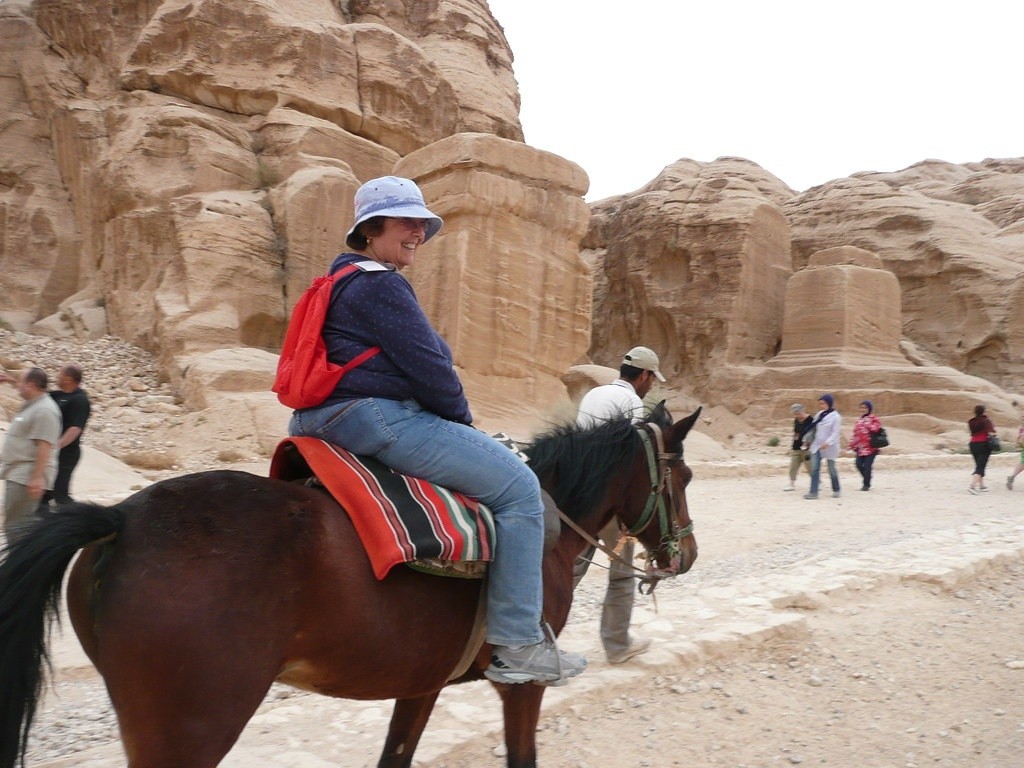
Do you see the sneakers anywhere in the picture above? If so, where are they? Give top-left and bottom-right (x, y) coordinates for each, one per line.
(484, 635), (588, 684)
(609, 637), (651, 663)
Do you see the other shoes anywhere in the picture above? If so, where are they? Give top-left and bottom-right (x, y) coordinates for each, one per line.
(833, 491), (839, 498)
(967, 485), (978, 495)
(1007, 476), (1014, 490)
(861, 482), (870, 491)
(783, 485), (795, 491)
(979, 486), (988, 492)
(804, 492), (818, 499)
(818, 483), (824, 489)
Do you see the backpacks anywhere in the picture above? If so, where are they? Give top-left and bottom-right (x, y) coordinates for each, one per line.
(271, 262), (382, 409)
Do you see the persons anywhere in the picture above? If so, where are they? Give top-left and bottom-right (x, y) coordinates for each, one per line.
(801, 394), (840, 500)
(572, 345), (653, 664)
(968, 405), (996, 495)
(287, 176), (587, 685)
(783, 403), (824, 491)
(0, 367), (62, 554)
(0, 364), (90, 506)
(1006, 425), (1024, 490)
(847, 400), (880, 491)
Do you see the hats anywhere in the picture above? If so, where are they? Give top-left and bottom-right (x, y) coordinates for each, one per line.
(861, 401), (872, 412)
(820, 394), (832, 408)
(345, 176), (443, 252)
(791, 404), (804, 414)
(623, 347), (666, 383)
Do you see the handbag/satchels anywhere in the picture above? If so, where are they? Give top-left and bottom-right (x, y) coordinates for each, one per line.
(987, 438), (1000, 451)
(871, 428), (889, 448)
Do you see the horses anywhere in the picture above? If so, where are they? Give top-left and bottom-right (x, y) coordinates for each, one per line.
(0, 398), (704, 768)
